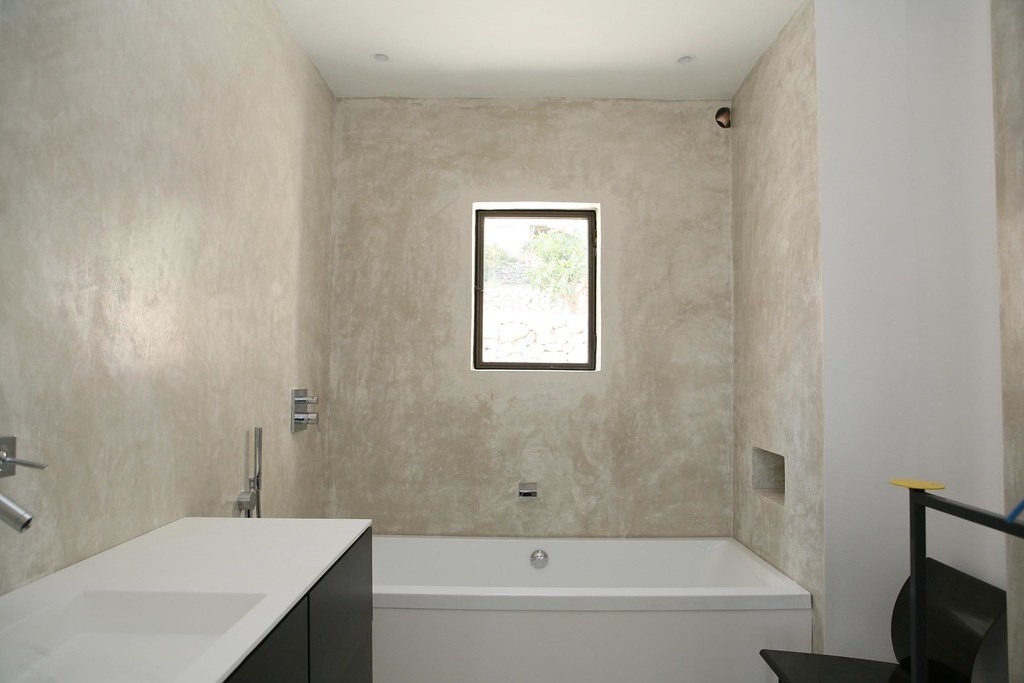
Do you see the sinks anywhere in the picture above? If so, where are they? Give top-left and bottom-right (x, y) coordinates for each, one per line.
(0, 588), (267, 683)
(111, 526), (331, 585)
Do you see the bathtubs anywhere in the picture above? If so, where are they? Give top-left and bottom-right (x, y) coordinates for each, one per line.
(373, 536), (811, 683)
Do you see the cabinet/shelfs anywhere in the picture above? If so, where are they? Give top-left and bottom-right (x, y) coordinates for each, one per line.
(225, 525), (373, 683)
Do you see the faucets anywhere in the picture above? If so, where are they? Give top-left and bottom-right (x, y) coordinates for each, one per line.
(0, 494), (34, 532)
